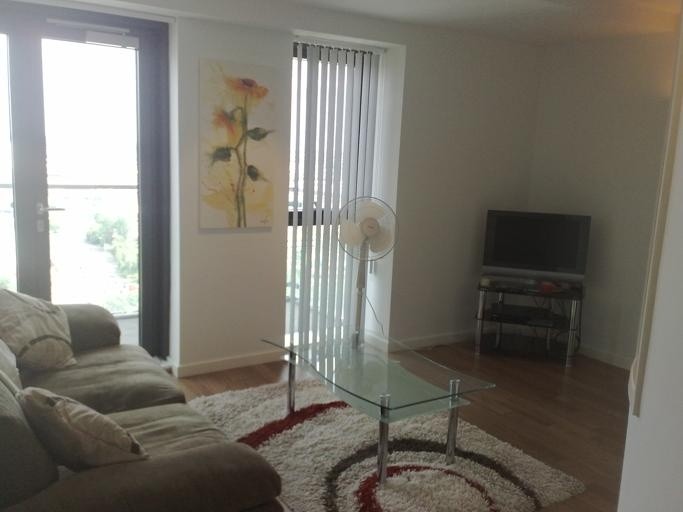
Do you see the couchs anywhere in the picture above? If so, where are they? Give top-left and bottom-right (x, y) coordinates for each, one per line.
(0, 287), (285, 512)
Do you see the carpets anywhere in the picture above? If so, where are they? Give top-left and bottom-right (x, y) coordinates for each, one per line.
(188, 375), (585, 512)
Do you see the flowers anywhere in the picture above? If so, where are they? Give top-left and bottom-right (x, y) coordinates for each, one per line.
(200, 76), (276, 229)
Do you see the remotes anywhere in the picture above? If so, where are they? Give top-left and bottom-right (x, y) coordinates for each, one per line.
(495, 287), (528, 294)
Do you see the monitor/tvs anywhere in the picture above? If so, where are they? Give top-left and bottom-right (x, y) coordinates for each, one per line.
(480, 209), (591, 293)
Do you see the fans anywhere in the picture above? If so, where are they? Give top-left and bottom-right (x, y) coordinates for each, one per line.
(334, 195), (400, 332)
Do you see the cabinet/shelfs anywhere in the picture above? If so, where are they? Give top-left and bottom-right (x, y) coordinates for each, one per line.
(471, 280), (584, 368)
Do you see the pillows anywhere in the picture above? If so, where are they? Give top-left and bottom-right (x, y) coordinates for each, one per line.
(0, 288), (78, 371)
(16, 385), (149, 472)
